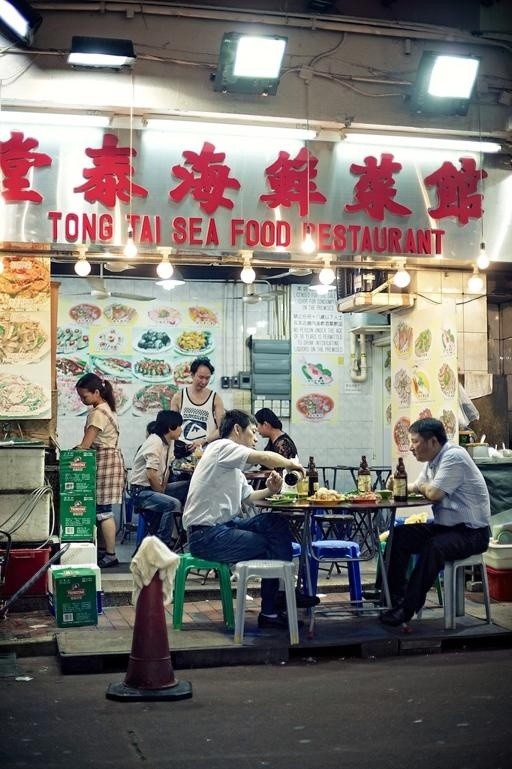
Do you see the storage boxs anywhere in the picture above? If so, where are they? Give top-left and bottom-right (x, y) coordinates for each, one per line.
(0, 449), (103, 627)
(481, 541), (512, 602)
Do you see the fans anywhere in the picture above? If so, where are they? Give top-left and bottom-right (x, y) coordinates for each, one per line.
(56, 263), (155, 302)
(217, 269), (286, 304)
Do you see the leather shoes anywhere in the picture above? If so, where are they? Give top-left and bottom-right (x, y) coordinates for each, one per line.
(258, 589), (320, 629)
(362, 588), (418, 626)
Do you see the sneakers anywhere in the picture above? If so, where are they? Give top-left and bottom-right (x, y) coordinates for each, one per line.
(97, 557), (119, 568)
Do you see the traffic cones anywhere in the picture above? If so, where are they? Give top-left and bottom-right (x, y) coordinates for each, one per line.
(103, 532), (195, 703)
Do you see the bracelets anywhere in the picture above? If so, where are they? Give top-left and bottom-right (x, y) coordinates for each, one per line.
(202, 436), (210, 443)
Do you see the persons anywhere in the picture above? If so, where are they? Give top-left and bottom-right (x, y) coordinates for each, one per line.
(131, 422), (203, 483)
(170, 355), (223, 468)
(130, 411), (194, 552)
(183, 408), (320, 630)
(248, 408), (299, 491)
(76, 373), (122, 566)
(375, 418), (489, 627)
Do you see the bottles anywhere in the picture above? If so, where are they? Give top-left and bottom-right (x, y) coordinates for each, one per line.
(306, 455), (318, 497)
(285, 469), (304, 485)
(358, 455), (371, 491)
(458, 429), (477, 452)
(394, 458), (408, 502)
(195, 444), (203, 469)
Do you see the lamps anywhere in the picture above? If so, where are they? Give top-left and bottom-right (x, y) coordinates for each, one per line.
(210, 31), (288, 95)
(124, 63), (137, 259)
(64, 36), (136, 70)
(0, 0), (44, 47)
(407, 50), (482, 117)
(466, 262), (485, 295)
(299, 65), (317, 254)
(475, 54), (490, 268)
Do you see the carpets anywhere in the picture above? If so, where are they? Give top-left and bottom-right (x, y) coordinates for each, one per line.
(427, 587), (512, 631)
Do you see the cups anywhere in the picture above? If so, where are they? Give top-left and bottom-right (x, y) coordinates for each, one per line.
(296, 477), (309, 500)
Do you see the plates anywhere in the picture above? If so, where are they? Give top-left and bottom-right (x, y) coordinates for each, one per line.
(0, 256), (221, 418)
(265, 490), (425, 504)
(297, 363), (336, 422)
(384, 325), (458, 455)
